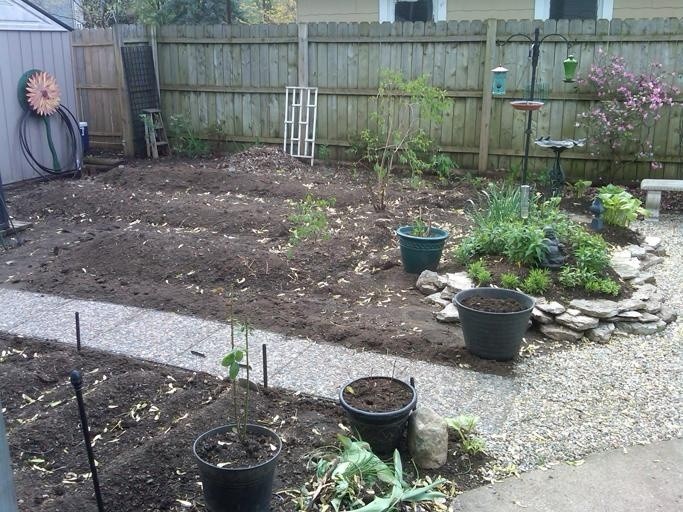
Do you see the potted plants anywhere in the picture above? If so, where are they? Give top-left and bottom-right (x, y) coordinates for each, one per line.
(397, 217), (449, 274)
(193, 279), (283, 512)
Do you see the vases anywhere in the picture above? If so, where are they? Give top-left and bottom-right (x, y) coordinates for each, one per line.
(341, 375), (417, 456)
(454, 287), (534, 362)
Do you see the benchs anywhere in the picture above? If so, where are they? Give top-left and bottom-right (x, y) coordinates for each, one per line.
(641, 178), (683, 221)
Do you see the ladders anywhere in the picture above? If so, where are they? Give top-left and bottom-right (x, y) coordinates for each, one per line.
(142, 108), (173, 158)
